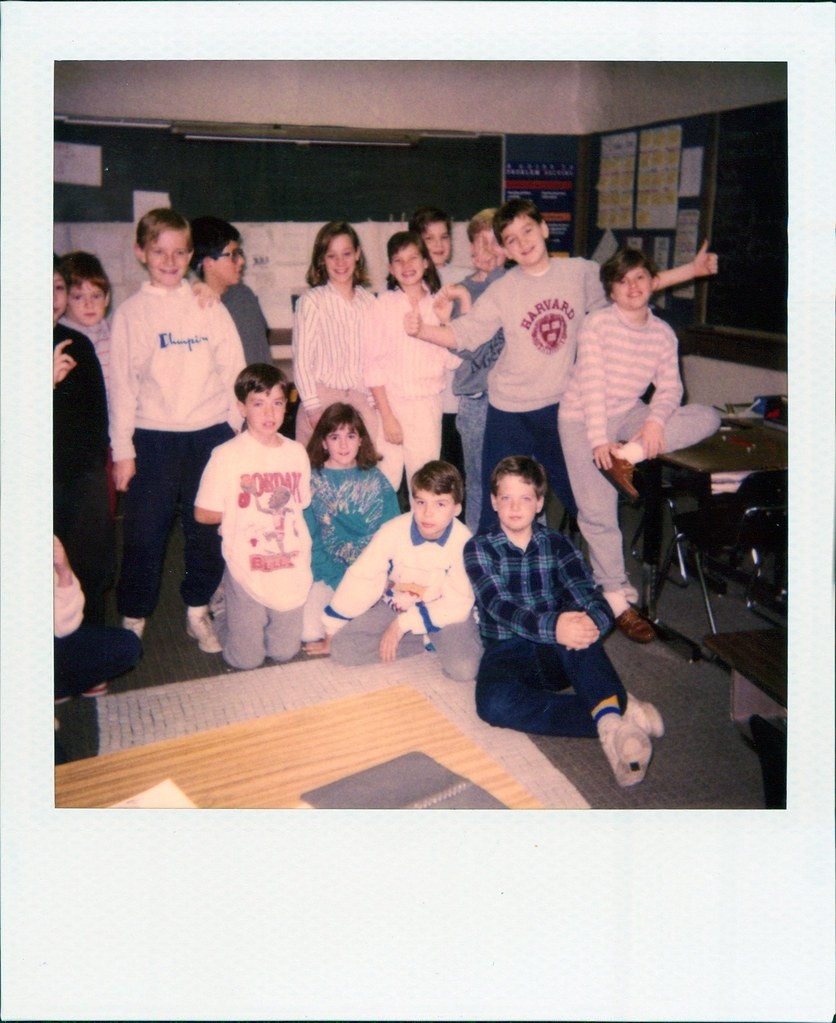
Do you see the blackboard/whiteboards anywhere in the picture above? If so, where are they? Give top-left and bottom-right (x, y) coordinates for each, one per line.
(53, 112), (506, 347)
(692, 95), (788, 342)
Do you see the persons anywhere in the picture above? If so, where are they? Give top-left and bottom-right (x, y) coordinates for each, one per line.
(364, 210), (510, 536)
(53, 251), (145, 704)
(302, 402), (402, 641)
(292, 222), (375, 446)
(107, 207), (248, 652)
(463, 456), (664, 788)
(558, 250), (721, 642)
(194, 363), (313, 668)
(403, 203), (718, 560)
(189, 215), (272, 364)
(302, 460), (483, 681)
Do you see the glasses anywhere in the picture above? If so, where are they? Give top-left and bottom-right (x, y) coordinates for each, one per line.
(196, 247), (243, 272)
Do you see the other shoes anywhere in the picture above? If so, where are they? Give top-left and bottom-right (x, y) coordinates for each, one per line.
(593, 452), (638, 500)
(602, 719), (652, 787)
(621, 692), (664, 738)
(614, 607), (656, 644)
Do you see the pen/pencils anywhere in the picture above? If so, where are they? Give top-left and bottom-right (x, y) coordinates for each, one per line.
(713, 405), (727, 413)
(725, 403), (731, 414)
(728, 436), (756, 447)
(730, 404), (736, 414)
(745, 400), (761, 413)
(719, 427), (738, 432)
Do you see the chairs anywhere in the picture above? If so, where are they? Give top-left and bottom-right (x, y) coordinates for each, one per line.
(652, 468), (788, 663)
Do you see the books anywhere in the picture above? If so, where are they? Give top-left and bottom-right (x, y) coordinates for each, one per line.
(300, 751), (509, 809)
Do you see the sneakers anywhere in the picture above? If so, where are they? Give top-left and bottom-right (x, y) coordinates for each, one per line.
(123, 617), (146, 638)
(185, 613), (222, 652)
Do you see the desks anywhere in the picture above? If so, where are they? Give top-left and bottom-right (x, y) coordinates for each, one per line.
(631, 417), (788, 663)
